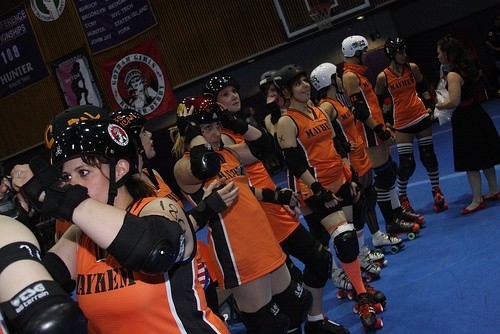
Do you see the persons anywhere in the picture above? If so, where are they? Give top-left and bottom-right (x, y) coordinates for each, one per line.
(372, 15), (500, 215)
(108, 76), (350, 334)
(264, 62), (405, 334)
(342, 35), (426, 240)
(0, 104), (232, 334)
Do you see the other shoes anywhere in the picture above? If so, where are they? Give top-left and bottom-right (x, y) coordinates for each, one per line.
(460, 200), (487, 214)
(482, 191), (500, 200)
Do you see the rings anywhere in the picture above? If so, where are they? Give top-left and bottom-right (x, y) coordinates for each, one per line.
(17, 169), (25, 178)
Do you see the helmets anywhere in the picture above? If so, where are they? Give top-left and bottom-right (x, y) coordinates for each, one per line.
(384, 37), (408, 59)
(309, 62), (338, 92)
(259, 69), (280, 91)
(176, 94), (222, 137)
(108, 109), (148, 132)
(49, 118), (137, 170)
(273, 64), (306, 98)
(341, 35), (370, 58)
(42, 105), (112, 151)
(206, 75), (241, 103)
(73, 63), (80, 67)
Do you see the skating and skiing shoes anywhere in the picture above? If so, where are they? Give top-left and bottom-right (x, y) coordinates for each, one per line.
(357, 297), (384, 331)
(331, 268), (356, 300)
(371, 232), (404, 253)
(360, 251), (381, 282)
(387, 217), (420, 240)
(304, 316), (350, 334)
(393, 204), (425, 223)
(433, 187), (448, 213)
(352, 285), (387, 314)
(359, 244), (388, 265)
